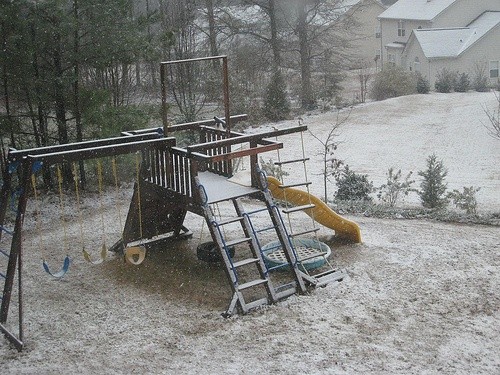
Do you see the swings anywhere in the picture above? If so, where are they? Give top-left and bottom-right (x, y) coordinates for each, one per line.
(72, 157), (107, 264)
(32, 163), (70, 278)
(111, 151), (147, 266)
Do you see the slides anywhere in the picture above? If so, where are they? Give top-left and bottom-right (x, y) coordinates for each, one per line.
(250, 170), (361, 243)
